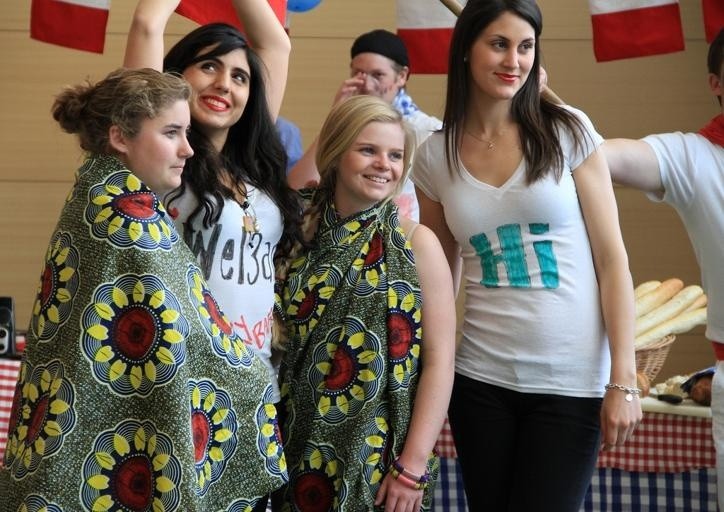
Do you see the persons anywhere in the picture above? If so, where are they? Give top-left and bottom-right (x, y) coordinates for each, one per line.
(289, 28), (445, 232)
(122, 1), (291, 408)
(411, 2), (641, 512)
(261, 95), (465, 511)
(1, 66), (289, 512)
(533, 19), (724, 511)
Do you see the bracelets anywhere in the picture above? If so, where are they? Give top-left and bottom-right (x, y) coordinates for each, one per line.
(388, 459), (430, 490)
(602, 381), (644, 399)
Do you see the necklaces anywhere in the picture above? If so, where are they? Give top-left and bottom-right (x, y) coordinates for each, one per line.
(231, 192), (262, 234)
(464, 118), (519, 149)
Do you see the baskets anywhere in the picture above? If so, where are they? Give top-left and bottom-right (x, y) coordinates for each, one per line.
(636, 334), (675, 389)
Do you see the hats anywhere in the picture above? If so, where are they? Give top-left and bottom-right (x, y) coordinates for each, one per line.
(351, 30), (408, 66)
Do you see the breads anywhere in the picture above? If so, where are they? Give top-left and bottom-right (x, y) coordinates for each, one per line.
(636, 279), (708, 347)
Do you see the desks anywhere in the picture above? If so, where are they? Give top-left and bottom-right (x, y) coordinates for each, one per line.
(0, 361), (719, 512)
